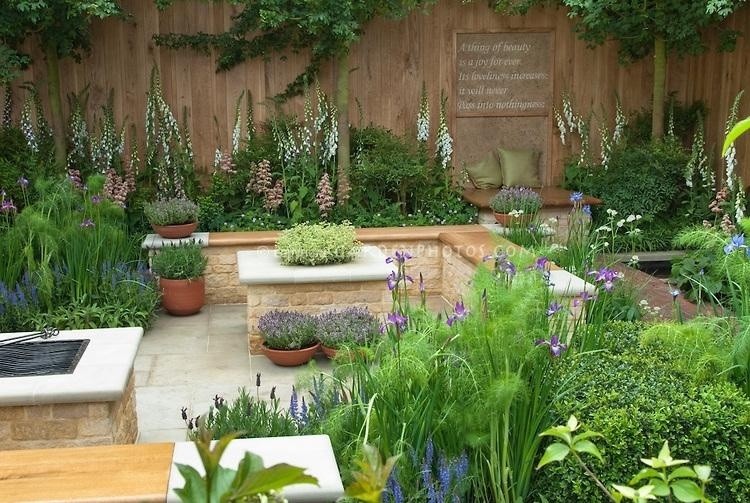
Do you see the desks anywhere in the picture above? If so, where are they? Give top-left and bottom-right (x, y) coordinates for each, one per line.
(1, 326), (145, 445)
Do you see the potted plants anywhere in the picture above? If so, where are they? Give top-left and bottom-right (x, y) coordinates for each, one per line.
(148, 238), (209, 317)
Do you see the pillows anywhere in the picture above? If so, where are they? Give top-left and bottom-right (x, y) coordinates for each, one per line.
(497, 146), (545, 189)
(461, 151), (501, 192)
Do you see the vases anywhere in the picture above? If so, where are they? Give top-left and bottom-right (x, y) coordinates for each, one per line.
(321, 345), (375, 362)
(153, 225), (195, 241)
(498, 212), (528, 225)
(260, 339), (319, 366)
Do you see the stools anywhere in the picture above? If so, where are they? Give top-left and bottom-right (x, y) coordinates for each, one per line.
(164, 433), (346, 503)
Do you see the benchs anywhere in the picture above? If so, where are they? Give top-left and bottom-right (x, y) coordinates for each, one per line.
(1, 444), (173, 500)
(452, 184), (602, 243)
(436, 230), (603, 358)
(141, 226), (599, 342)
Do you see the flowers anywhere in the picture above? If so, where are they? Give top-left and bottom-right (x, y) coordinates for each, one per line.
(488, 183), (543, 216)
(256, 308), (320, 348)
(309, 306), (383, 346)
(143, 195), (201, 223)
